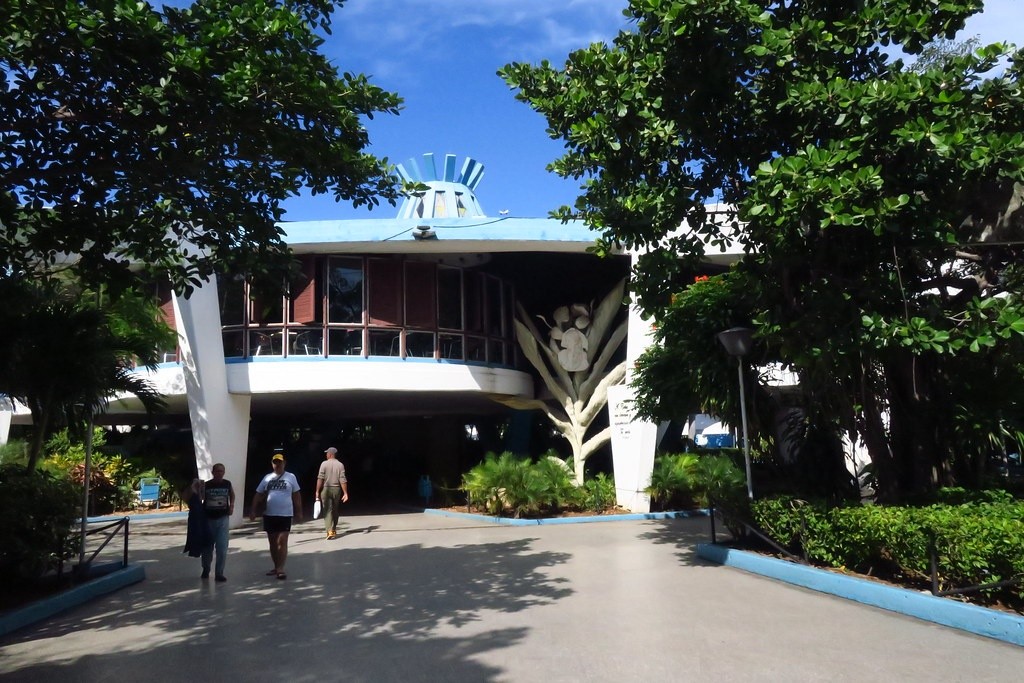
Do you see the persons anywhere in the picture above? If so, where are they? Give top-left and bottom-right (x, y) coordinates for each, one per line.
(249, 454), (302, 579)
(316, 447), (349, 540)
(185, 463), (235, 582)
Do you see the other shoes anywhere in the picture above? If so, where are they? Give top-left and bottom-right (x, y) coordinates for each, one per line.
(215, 575), (227, 581)
(200, 572), (210, 578)
(277, 571), (287, 579)
(265, 567), (282, 576)
(326, 530), (336, 540)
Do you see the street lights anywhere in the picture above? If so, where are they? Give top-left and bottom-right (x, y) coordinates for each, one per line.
(720, 326), (755, 502)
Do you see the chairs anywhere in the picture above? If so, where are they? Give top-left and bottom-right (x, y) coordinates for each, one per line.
(253, 328), (481, 359)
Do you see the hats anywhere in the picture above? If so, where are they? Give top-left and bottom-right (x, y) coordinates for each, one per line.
(272, 454), (284, 461)
(324, 447), (337, 453)
